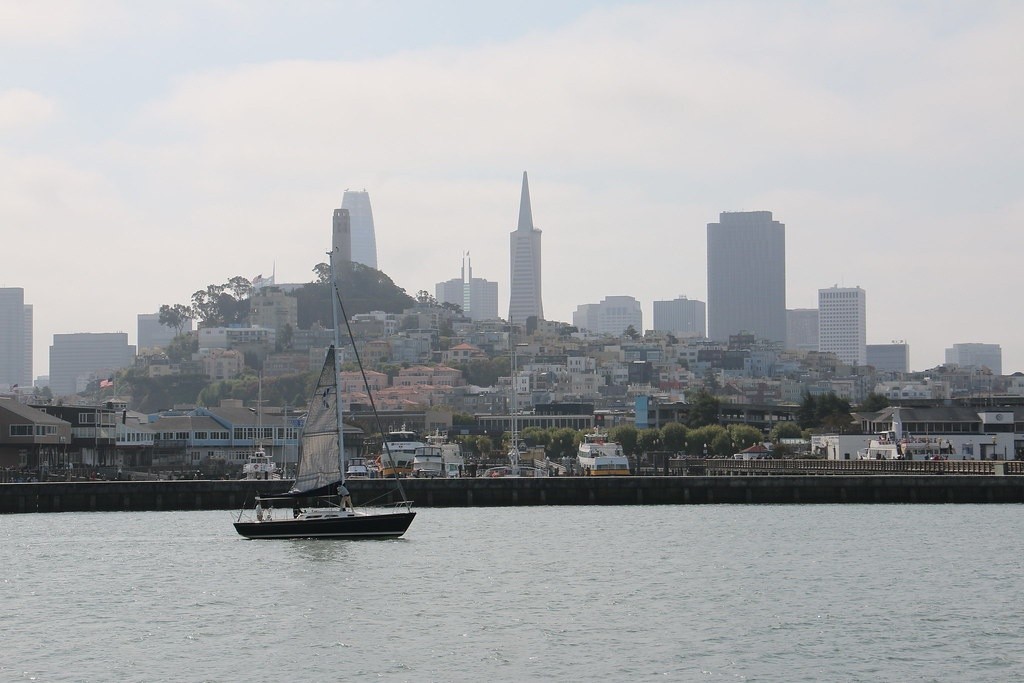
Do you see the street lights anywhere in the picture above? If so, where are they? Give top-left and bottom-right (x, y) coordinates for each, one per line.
(992, 435), (996, 455)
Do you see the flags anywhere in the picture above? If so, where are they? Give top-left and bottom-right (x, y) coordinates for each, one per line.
(982, 366), (994, 376)
(8, 384), (18, 392)
(100, 377), (114, 388)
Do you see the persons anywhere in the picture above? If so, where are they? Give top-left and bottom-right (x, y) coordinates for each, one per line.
(256, 501), (263, 522)
(0, 465), (206, 483)
(370, 464), (711, 478)
(335, 483), (355, 514)
(292, 500), (302, 519)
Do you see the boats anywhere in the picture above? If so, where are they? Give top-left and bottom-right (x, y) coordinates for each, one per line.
(346, 456), (370, 481)
(412, 428), (464, 477)
(380, 423), (424, 478)
(578, 425), (632, 476)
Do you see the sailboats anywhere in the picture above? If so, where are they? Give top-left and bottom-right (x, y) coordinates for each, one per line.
(482, 316), (550, 476)
(232, 251), (418, 540)
(238, 371), (288, 480)
